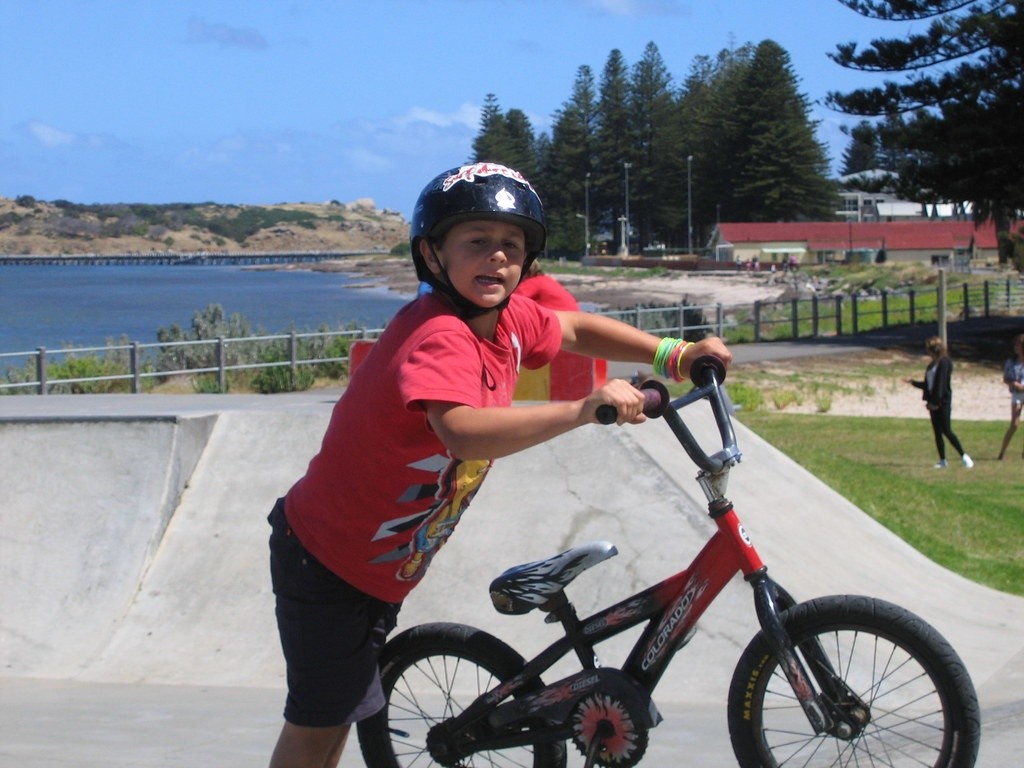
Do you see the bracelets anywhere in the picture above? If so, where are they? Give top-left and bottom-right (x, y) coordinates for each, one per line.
(653, 337), (697, 382)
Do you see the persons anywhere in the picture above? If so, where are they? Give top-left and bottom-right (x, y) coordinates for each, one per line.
(267, 160), (734, 768)
(782, 254), (799, 272)
(899, 337), (978, 470)
(745, 254), (760, 271)
(998, 335), (1024, 461)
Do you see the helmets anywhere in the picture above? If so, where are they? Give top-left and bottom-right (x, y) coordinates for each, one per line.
(410, 162), (546, 287)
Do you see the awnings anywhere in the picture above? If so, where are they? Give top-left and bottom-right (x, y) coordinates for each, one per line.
(762, 248), (807, 253)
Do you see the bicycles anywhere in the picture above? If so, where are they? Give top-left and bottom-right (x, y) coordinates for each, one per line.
(357, 354), (981, 768)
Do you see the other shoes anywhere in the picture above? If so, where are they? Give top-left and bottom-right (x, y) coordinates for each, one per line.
(934, 460), (947, 468)
(961, 453), (973, 468)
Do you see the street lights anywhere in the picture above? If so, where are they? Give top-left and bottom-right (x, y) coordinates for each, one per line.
(585, 156), (693, 256)
